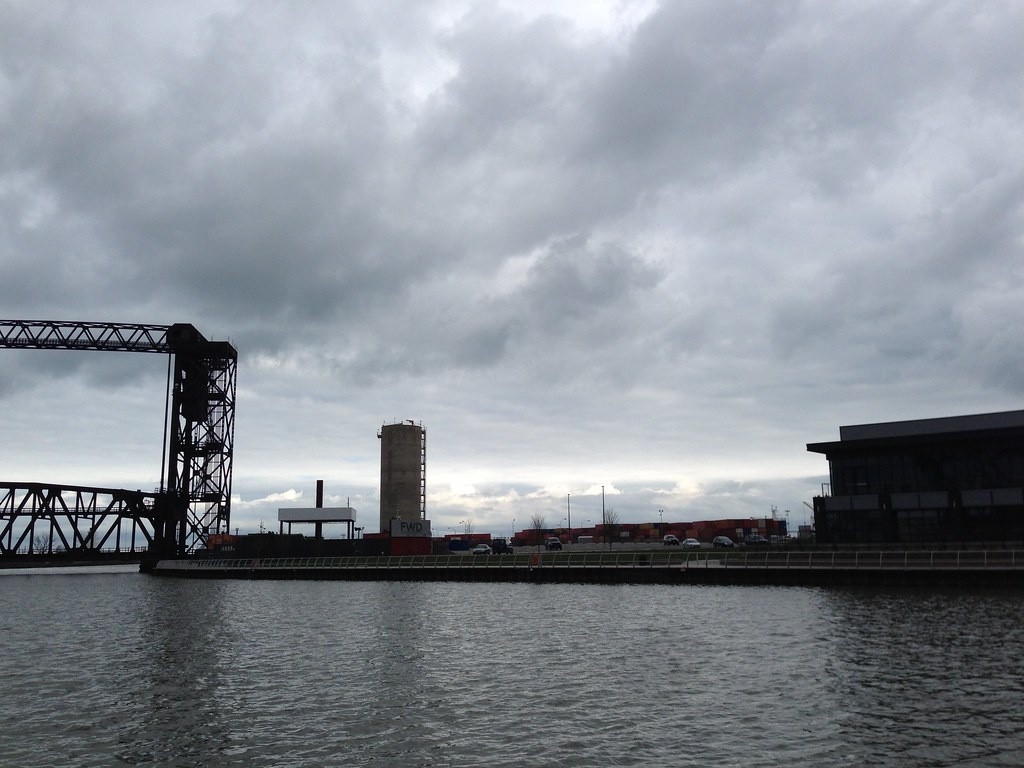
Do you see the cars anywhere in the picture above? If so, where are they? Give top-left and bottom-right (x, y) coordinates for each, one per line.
(682, 537), (702, 549)
(544, 537), (562, 550)
(745, 534), (769, 545)
(712, 535), (735, 549)
(473, 544), (491, 556)
(766, 534), (791, 545)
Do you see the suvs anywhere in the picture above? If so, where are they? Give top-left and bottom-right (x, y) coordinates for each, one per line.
(664, 535), (679, 545)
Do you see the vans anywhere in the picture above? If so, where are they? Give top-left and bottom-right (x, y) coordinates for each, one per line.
(492, 538), (513, 555)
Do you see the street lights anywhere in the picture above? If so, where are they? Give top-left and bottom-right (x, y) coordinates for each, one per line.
(568, 494), (572, 544)
(560, 518), (567, 528)
(432, 529), (439, 537)
(601, 485), (606, 544)
(448, 527), (456, 534)
(580, 519), (590, 528)
(459, 521), (467, 533)
(512, 519), (516, 536)
(658, 509), (664, 523)
